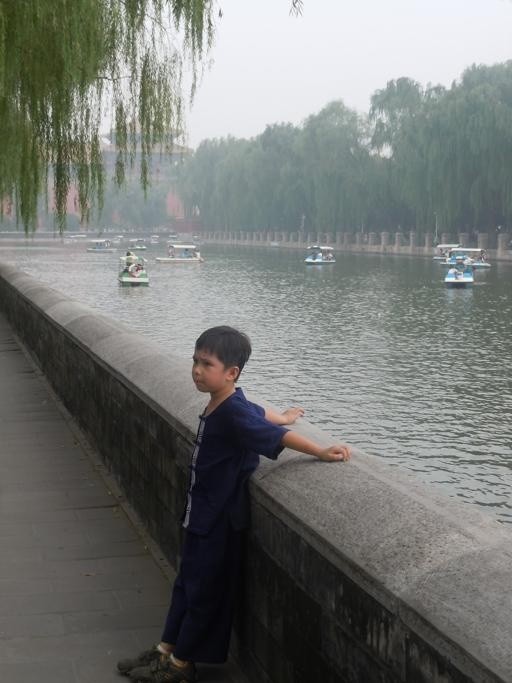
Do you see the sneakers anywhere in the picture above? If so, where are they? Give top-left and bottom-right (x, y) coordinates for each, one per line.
(116, 643), (166, 675)
(128, 654), (198, 682)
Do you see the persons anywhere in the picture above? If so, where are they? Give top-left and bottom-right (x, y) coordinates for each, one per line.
(182, 249), (193, 258)
(437, 247), (486, 277)
(311, 250), (335, 261)
(116, 323), (352, 682)
(123, 250), (144, 276)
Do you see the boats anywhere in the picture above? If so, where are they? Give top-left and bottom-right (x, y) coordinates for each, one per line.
(154, 242), (203, 264)
(115, 251), (151, 288)
(67, 231), (201, 255)
(303, 244), (336, 266)
(431, 241), (494, 289)
